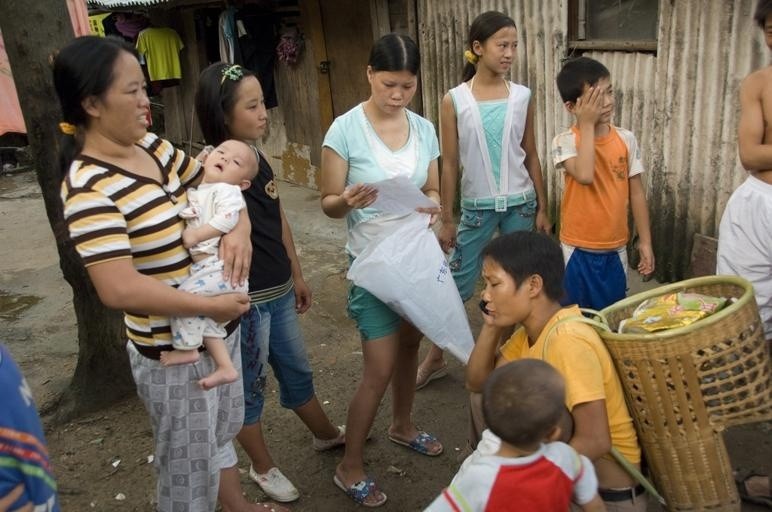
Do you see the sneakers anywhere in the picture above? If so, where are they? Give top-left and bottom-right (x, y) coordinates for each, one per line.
(313, 425), (373, 451)
(249, 463), (300, 502)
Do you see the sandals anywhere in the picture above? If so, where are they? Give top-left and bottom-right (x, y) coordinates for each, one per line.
(733, 465), (772, 508)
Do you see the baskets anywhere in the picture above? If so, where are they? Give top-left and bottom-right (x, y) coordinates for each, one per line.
(591, 275), (772, 512)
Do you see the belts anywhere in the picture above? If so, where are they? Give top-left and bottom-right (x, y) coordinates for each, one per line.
(597, 483), (645, 502)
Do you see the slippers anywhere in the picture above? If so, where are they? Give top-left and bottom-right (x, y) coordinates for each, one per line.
(333, 474), (388, 507)
(414, 360), (448, 391)
(389, 429), (443, 456)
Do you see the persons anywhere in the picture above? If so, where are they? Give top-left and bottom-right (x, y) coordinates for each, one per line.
(546, 56), (658, 317)
(51, 33), (298, 512)
(181, 58), (377, 505)
(463, 228), (651, 510)
(421, 355), (610, 511)
(158, 134), (266, 391)
(317, 34), (446, 512)
(713, 2), (772, 348)
(415, 9), (554, 394)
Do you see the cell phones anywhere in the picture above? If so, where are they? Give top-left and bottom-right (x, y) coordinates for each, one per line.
(479, 298), (491, 319)
